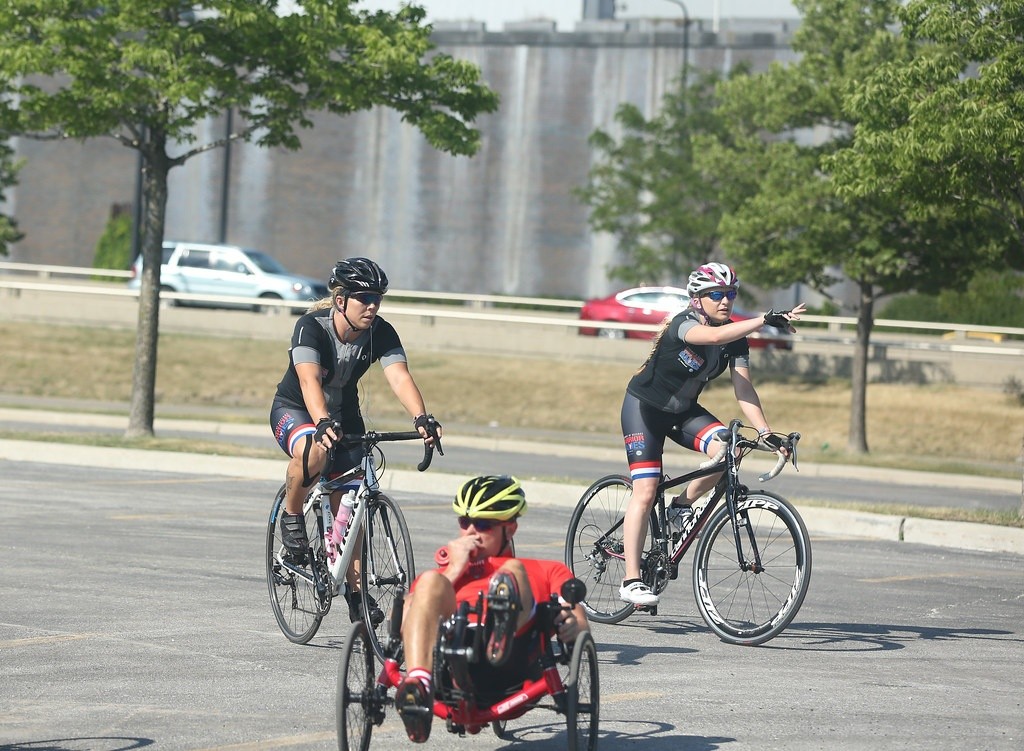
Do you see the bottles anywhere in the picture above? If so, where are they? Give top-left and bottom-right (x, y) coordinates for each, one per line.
(324, 527), (337, 562)
(332, 489), (355, 544)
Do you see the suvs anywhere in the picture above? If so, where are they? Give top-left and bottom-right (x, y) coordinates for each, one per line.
(129, 239), (331, 318)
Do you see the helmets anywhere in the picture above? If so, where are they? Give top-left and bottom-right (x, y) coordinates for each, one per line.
(328, 257), (389, 294)
(453, 474), (528, 521)
(686, 262), (740, 297)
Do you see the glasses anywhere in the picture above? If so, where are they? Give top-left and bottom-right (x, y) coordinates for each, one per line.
(459, 516), (515, 532)
(335, 293), (383, 305)
(694, 290), (736, 300)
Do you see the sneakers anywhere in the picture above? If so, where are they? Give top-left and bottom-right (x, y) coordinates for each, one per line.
(395, 678), (432, 743)
(667, 497), (702, 538)
(618, 581), (660, 606)
(485, 570), (520, 667)
(280, 508), (309, 552)
(350, 609), (385, 629)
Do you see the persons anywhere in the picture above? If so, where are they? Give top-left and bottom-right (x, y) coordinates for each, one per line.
(620, 262), (807, 606)
(270, 256), (442, 628)
(394, 474), (590, 744)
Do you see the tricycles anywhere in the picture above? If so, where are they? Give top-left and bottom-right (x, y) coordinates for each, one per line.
(336, 578), (600, 751)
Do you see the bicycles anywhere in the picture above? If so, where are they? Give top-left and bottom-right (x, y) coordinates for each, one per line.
(563, 420), (813, 648)
(267, 422), (446, 666)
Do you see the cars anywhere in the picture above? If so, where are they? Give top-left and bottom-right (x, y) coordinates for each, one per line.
(580, 285), (794, 351)
